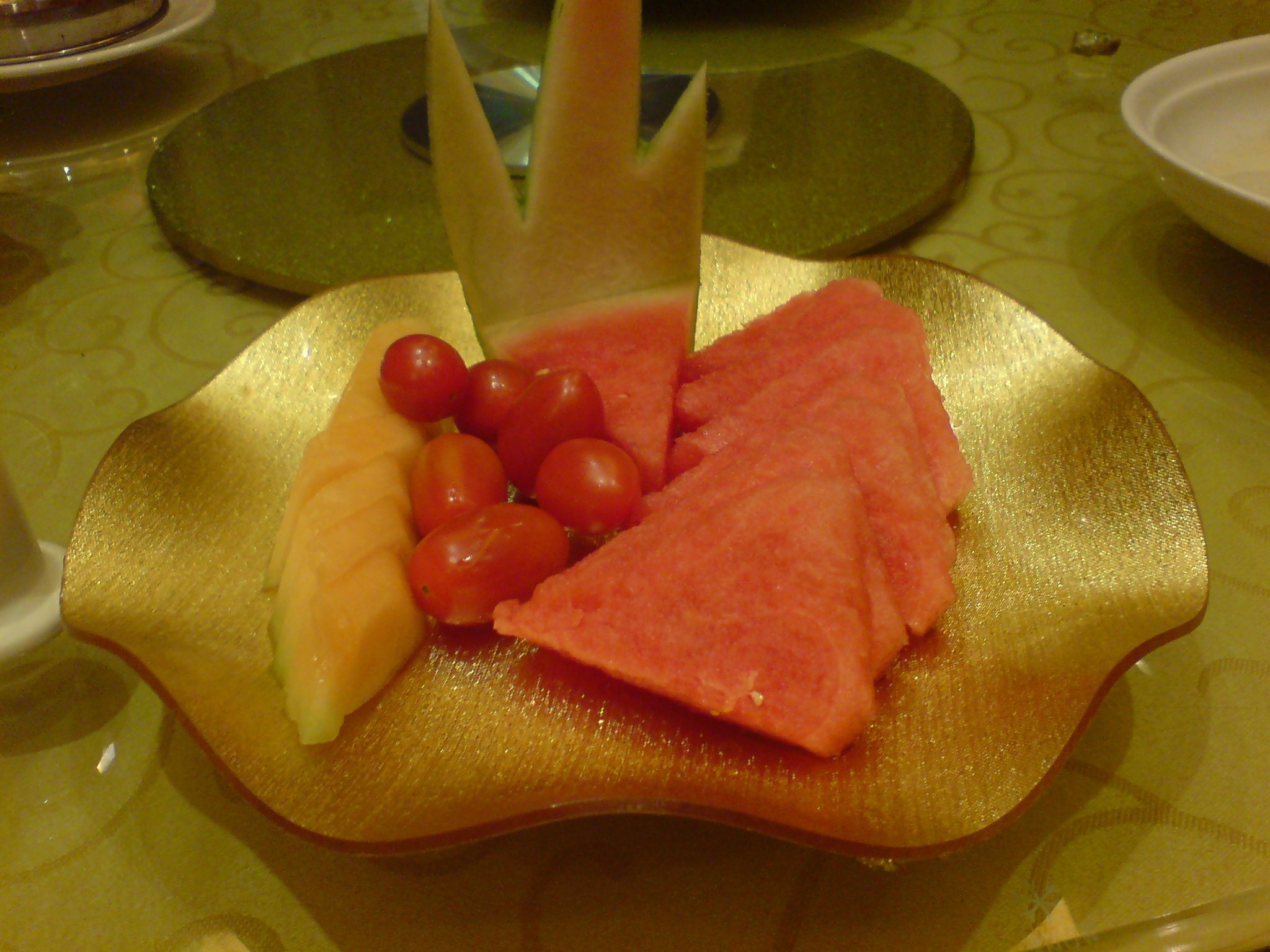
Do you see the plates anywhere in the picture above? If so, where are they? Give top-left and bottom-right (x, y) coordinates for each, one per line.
(0, 42), (269, 192)
(57, 233), (1212, 861)
(0, 1), (221, 96)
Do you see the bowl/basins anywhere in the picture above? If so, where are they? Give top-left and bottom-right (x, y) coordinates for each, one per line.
(0, 1), (170, 67)
(1120, 35), (1269, 269)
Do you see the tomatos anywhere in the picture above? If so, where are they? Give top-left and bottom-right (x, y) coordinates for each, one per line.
(377, 334), (643, 626)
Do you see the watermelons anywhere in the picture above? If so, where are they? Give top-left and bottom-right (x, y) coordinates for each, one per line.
(491, 269), (972, 753)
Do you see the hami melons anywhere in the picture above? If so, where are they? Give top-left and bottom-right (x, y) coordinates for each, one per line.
(271, 316), (440, 746)
